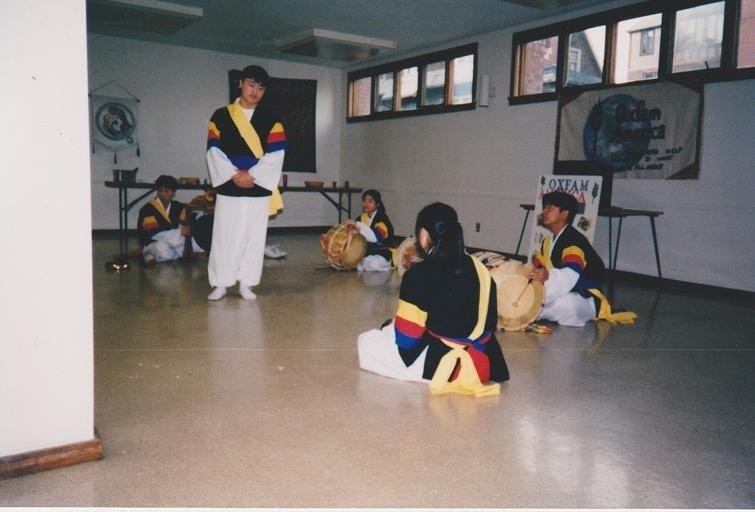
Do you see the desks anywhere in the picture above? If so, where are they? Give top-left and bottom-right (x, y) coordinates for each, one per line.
(514, 202), (665, 299)
(103, 179), (364, 265)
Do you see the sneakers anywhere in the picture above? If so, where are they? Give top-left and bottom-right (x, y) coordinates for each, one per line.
(263, 244), (289, 259)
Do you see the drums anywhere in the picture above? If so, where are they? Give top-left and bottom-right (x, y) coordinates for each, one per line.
(480, 254), (544, 332)
(398, 236), (418, 276)
(471, 250), (504, 269)
(321, 224), (367, 272)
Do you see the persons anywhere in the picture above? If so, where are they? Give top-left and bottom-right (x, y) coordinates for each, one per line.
(345, 189), (395, 271)
(204, 63), (286, 302)
(132, 174), (214, 265)
(522, 190), (638, 334)
(353, 201), (511, 396)
